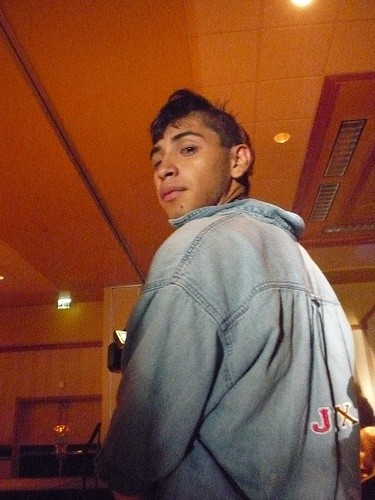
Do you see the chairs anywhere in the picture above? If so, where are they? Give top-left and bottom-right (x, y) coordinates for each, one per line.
(52, 422), (104, 489)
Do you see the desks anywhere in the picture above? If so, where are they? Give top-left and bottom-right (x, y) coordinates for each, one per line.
(17, 442), (101, 479)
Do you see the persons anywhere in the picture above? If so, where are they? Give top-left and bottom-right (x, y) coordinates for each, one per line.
(85, 86), (365, 500)
(355, 394), (375, 500)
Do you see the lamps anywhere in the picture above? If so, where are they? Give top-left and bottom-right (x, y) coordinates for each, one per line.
(56, 296), (72, 312)
